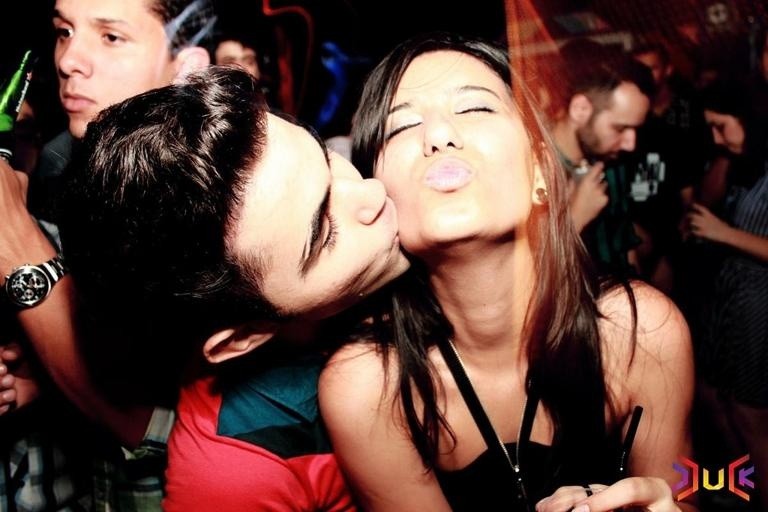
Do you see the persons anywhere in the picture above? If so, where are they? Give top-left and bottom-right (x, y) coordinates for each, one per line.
(0, 0), (768, 512)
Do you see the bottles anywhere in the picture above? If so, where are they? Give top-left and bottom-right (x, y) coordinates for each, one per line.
(1, 49), (38, 166)
